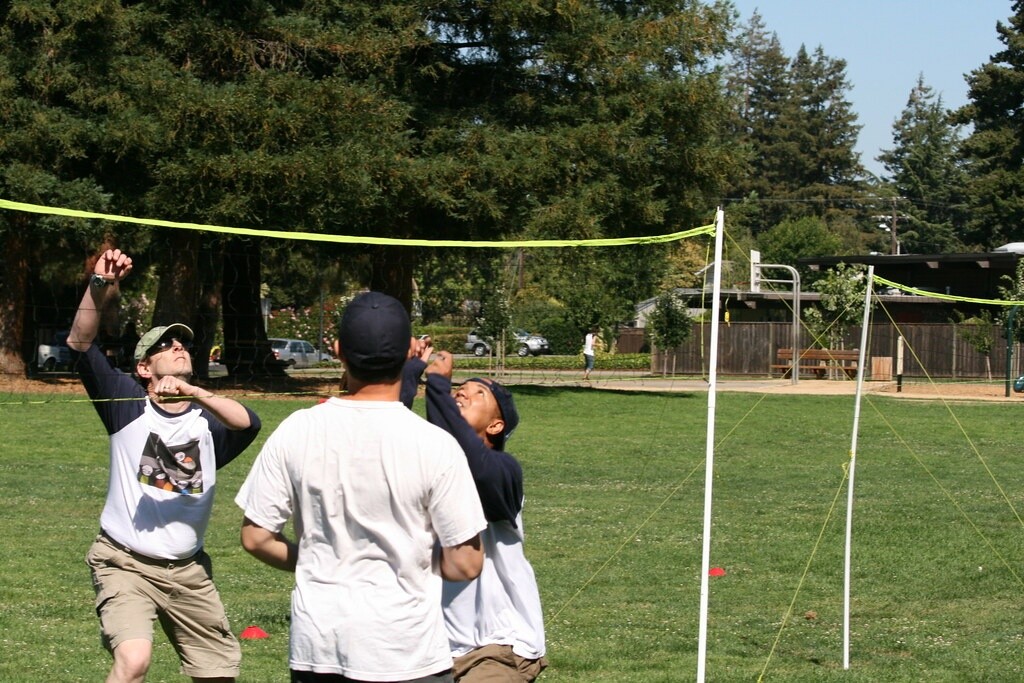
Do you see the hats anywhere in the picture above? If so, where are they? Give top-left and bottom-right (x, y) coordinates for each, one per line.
(340, 292), (410, 370)
(464, 377), (518, 452)
(134, 323), (194, 365)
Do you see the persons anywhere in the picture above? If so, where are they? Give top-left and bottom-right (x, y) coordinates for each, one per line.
(401, 336), (548, 683)
(62, 251), (261, 683)
(241, 290), (483, 683)
(583, 328), (605, 379)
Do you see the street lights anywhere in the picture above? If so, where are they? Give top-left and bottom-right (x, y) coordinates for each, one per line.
(878, 222), (901, 255)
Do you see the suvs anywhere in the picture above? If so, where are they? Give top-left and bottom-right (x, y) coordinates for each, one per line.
(464, 324), (549, 357)
(268, 337), (331, 369)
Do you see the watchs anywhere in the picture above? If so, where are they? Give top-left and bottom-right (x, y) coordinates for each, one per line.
(89, 274), (114, 289)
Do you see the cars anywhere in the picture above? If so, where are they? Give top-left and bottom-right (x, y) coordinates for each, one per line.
(37, 342), (73, 373)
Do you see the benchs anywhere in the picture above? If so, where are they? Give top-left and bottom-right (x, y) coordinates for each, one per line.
(770, 349), (868, 380)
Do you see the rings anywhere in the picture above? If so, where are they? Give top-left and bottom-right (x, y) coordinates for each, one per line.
(163, 386), (170, 389)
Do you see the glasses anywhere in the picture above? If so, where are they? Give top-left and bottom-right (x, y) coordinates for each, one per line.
(147, 336), (192, 357)
